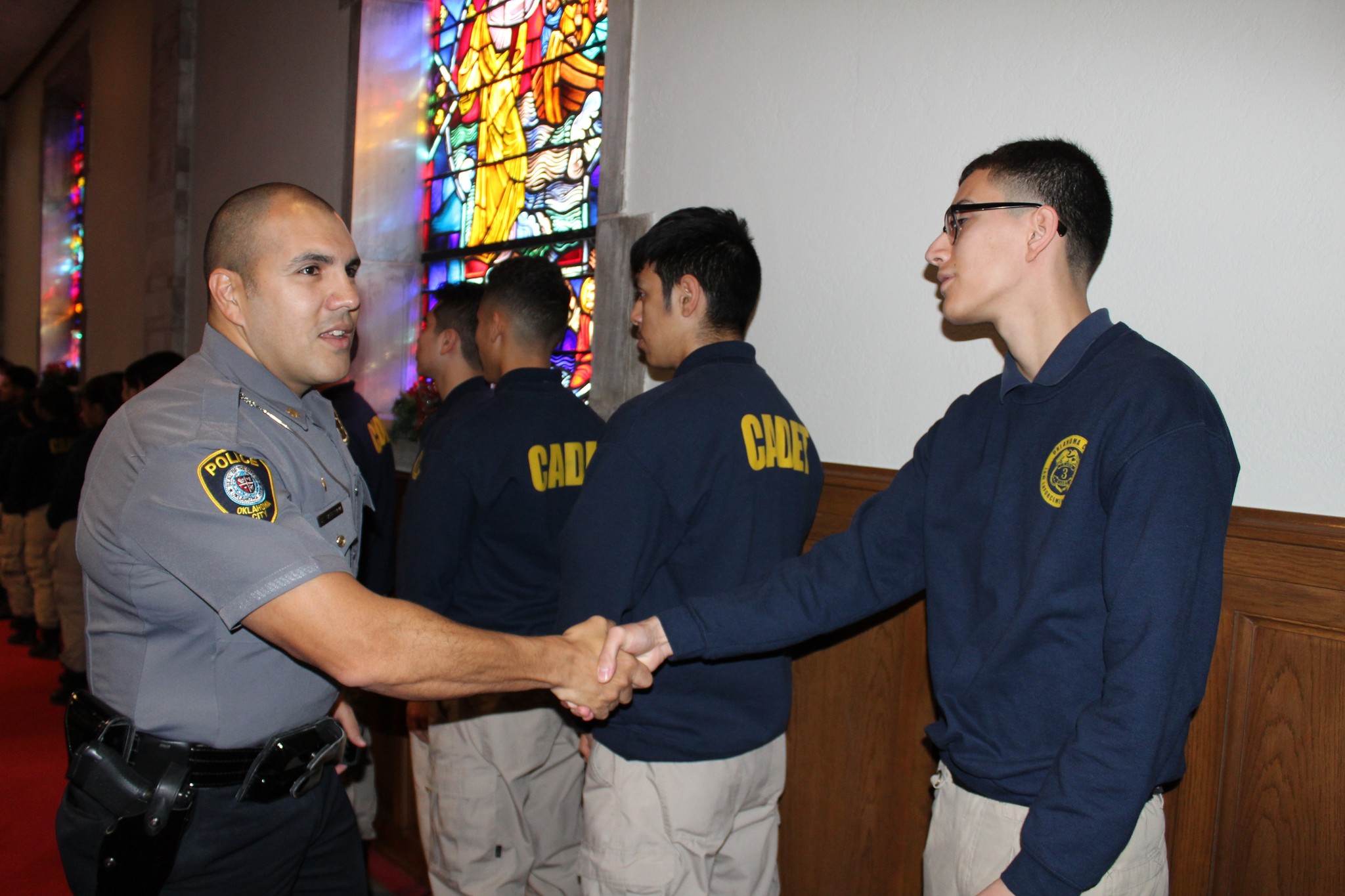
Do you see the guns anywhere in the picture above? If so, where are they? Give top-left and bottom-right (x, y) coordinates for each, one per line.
(64, 737), (198, 896)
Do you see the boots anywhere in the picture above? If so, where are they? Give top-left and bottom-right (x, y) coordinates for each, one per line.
(28, 624), (60, 660)
(8, 615), (34, 646)
(54, 670), (87, 703)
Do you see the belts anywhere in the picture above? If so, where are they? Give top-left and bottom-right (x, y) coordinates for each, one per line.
(86, 704), (266, 788)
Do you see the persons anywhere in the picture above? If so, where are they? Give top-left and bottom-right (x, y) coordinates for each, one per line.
(563, 137), (1244, 896)
(551, 205), (823, 896)
(402, 282), (494, 868)
(394, 255), (608, 895)
(313, 325), (405, 854)
(1, 351), (184, 705)
(54, 183), (654, 895)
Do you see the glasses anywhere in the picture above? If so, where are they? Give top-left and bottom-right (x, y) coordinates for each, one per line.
(943, 203), (1068, 245)
